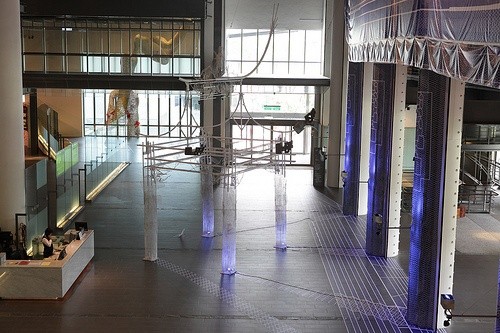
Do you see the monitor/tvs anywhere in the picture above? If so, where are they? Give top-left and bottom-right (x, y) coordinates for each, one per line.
(59, 246), (67, 260)
(75, 222), (87, 231)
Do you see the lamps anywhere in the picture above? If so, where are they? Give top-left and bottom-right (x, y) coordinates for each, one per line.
(341, 170), (348, 187)
(322, 146), (327, 159)
(374, 213), (383, 235)
(440, 293), (455, 325)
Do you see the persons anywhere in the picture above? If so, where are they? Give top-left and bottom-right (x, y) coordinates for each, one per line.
(41, 227), (54, 257)
